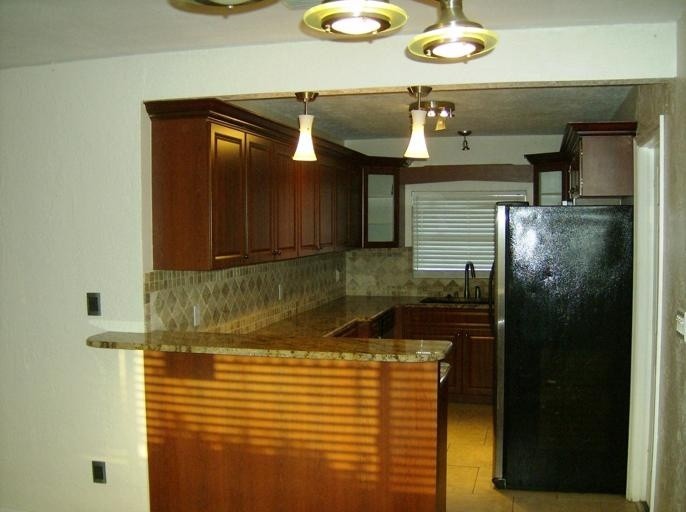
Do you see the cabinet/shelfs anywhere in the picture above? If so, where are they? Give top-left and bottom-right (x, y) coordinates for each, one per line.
(358, 310), (395, 339)
(568, 135), (632, 200)
(534, 162), (567, 206)
(151, 116), (400, 271)
(403, 310), (492, 407)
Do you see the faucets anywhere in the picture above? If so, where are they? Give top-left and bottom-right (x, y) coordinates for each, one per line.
(463, 261), (475, 298)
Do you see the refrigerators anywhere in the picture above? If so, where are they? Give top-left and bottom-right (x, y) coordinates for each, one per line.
(487, 202), (633, 494)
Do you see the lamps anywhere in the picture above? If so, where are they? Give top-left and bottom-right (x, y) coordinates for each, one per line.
(402, 86), (431, 159)
(291, 92), (320, 163)
(303, 1), (500, 59)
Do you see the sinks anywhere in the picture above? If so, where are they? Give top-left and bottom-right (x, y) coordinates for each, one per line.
(419, 296), (492, 305)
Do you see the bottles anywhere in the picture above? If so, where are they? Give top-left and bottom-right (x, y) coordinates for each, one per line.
(475, 285), (481, 300)
